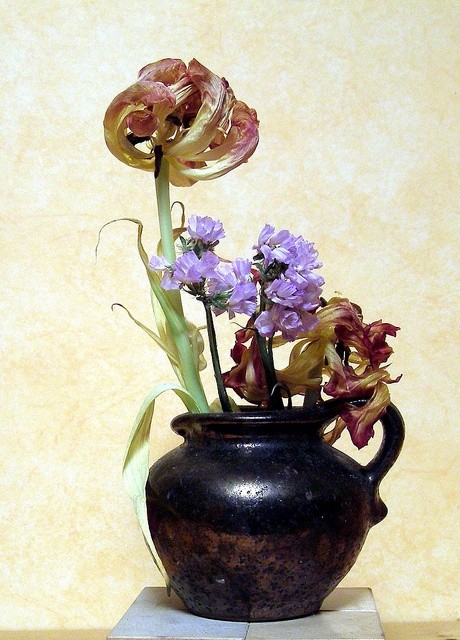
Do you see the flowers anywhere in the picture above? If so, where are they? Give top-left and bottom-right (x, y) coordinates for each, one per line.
(93, 54), (403, 597)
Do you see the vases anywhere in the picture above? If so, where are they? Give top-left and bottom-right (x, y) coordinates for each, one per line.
(143, 393), (407, 623)
